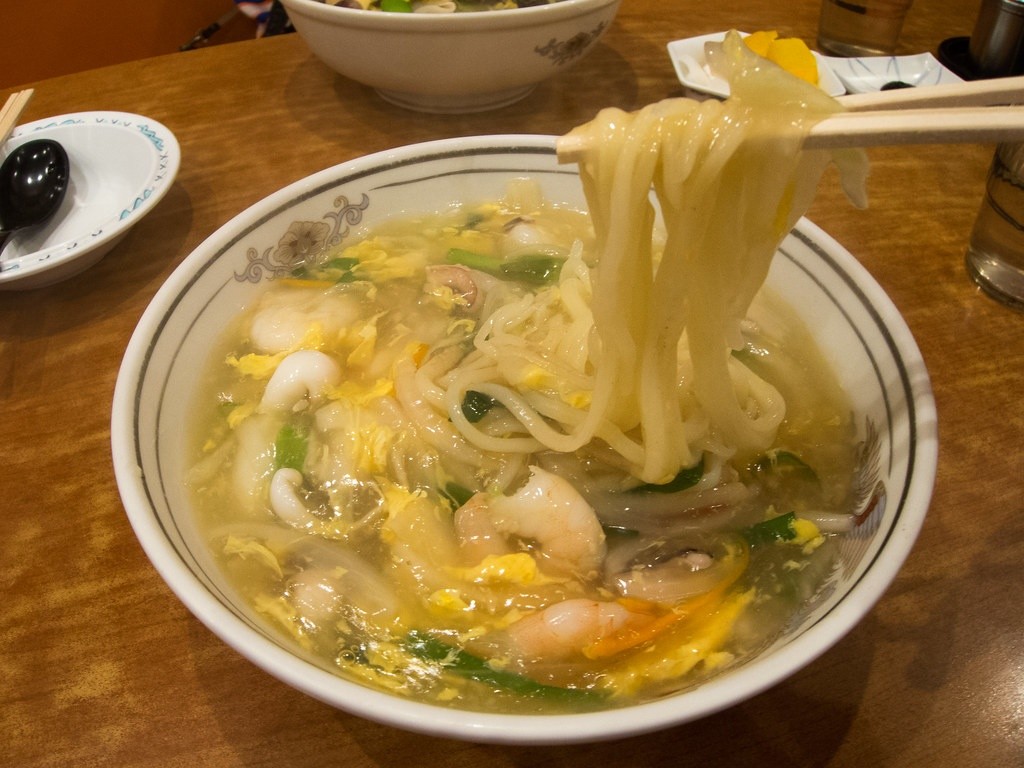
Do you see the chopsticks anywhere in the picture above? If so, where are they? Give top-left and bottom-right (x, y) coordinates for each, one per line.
(0, 87), (34, 146)
(556, 74), (1024, 167)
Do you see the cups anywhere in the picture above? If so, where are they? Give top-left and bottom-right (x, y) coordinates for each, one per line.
(965, 103), (1024, 316)
(965, 0), (1024, 81)
(817, 0), (909, 57)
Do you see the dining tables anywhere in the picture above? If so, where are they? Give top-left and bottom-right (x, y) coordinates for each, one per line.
(0, 0), (1024, 768)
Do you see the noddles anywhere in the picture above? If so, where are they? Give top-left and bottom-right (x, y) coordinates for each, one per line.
(208, 35), (862, 706)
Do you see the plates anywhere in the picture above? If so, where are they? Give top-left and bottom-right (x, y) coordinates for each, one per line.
(667, 30), (847, 101)
(825, 51), (966, 94)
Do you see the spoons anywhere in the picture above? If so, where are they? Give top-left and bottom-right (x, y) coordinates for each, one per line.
(1, 139), (71, 254)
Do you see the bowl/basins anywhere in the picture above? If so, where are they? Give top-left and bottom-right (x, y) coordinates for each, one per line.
(109, 128), (940, 745)
(0, 109), (182, 290)
(278, 0), (620, 116)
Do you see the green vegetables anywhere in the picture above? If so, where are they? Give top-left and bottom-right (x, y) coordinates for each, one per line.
(381, 0), (413, 12)
(275, 249), (823, 706)
